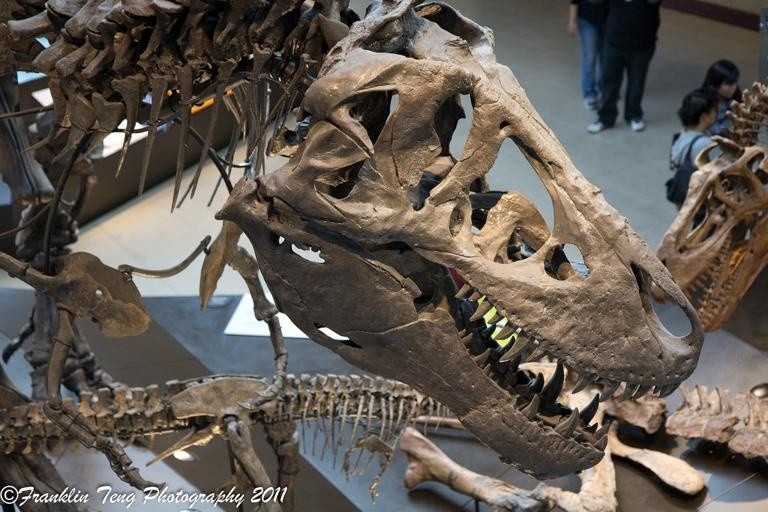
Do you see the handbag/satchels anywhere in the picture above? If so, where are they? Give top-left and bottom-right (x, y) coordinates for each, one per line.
(665, 134), (707, 204)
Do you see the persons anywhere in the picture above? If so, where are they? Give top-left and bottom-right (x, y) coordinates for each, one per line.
(567, 1), (662, 133)
(668, 58), (743, 234)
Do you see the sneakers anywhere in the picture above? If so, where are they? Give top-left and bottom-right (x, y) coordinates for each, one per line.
(587, 121), (644, 133)
